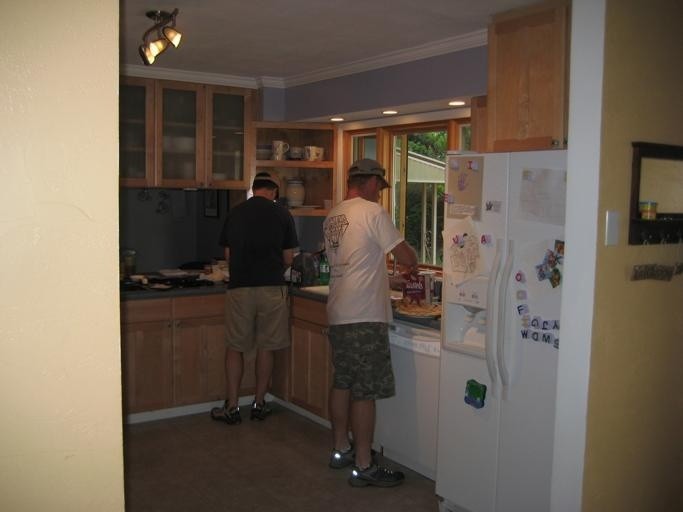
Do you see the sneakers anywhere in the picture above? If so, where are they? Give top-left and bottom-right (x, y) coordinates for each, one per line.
(348, 462), (405, 487)
(211, 400), (242, 425)
(249, 396), (272, 421)
(330, 442), (376, 468)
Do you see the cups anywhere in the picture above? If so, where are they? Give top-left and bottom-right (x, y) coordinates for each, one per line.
(637, 200), (657, 221)
(124, 251), (136, 282)
(322, 200), (331, 209)
(288, 148), (303, 158)
(269, 141), (289, 160)
(305, 145), (323, 160)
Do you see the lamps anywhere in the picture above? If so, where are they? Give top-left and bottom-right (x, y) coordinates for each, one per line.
(138, 8), (183, 66)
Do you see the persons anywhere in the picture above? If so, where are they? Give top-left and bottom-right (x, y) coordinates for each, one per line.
(323, 158), (418, 488)
(209, 170), (300, 424)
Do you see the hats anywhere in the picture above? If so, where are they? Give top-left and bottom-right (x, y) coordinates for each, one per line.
(254, 169), (280, 187)
(347, 159), (389, 190)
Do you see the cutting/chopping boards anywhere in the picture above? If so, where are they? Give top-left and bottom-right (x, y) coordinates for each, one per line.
(299, 285), (403, 302)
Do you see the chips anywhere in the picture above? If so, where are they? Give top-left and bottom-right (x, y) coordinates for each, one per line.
(397, 302), (443, 316)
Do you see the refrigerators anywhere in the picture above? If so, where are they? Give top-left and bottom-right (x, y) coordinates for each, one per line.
(434, 149), (570, 509)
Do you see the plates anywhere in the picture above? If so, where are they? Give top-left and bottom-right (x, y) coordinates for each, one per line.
(294, 205), (321, 209)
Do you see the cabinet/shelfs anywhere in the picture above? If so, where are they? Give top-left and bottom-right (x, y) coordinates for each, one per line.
(250, 120), (339, 217)
(486, 2), (568, 150)
(120, 72), (259, 188)
(121, 293), (351, 432)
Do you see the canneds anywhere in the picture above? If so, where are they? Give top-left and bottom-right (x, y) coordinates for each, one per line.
(639, 200), (658, 219)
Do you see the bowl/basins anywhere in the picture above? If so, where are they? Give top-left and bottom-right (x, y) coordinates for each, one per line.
(212, 173), (226, 180)
(171, 137), (194, 151)
(257, 145), (271, 160)
(162, 165), (183, 179)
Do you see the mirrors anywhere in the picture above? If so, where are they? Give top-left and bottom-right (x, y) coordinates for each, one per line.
(628, 141), (683, 244)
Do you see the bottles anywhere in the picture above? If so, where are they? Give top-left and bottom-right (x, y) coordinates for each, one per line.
(319, 256), (329, 283)
(286, 180), (305, 206)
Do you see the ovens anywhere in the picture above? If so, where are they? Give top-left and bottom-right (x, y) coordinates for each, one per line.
(369, 320), (441, 480)
